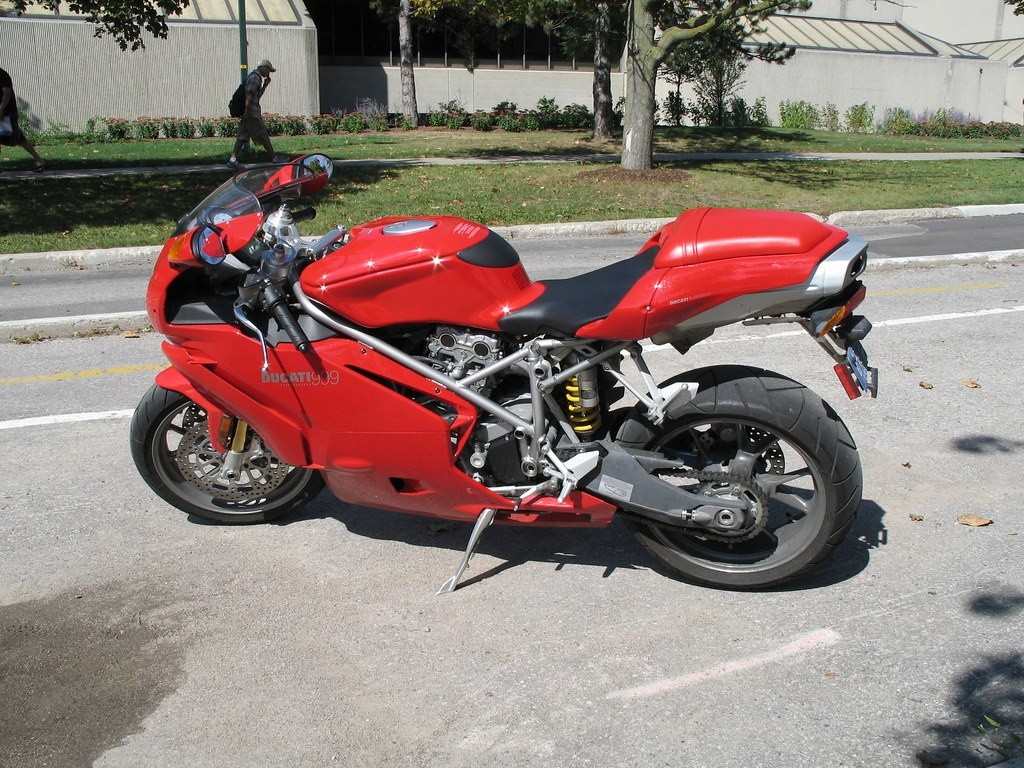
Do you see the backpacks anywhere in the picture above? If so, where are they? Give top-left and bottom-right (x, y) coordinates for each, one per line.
(227, 72), (262, 119)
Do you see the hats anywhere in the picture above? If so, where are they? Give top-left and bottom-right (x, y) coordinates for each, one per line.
(257, 59), (276, 73)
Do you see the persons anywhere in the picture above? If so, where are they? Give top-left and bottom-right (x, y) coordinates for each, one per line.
(0, 68), (47, 172)
(226, 61), (290, 170)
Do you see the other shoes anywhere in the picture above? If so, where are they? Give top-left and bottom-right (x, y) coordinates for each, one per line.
(34, 163), (49, 172)
(226, 159), (246, 171)
(272, 155), (291, 164)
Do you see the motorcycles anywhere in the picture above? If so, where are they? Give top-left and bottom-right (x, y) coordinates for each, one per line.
(130, 151), (880, 594)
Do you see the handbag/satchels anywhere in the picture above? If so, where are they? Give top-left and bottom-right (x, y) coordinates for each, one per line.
(0, 115), (14, 136)
